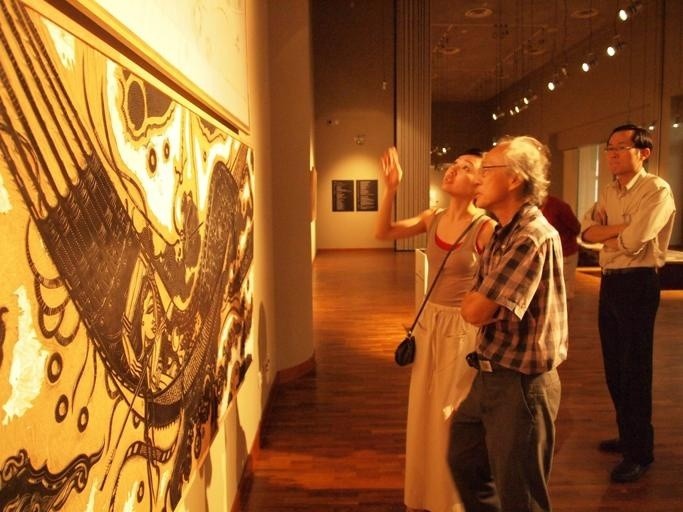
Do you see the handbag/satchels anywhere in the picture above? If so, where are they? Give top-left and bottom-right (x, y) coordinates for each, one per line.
(394, 335), (415, 366)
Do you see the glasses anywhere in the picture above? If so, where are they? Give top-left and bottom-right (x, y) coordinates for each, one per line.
(478, 165), (507, 178)
(604, 146), (635, 152)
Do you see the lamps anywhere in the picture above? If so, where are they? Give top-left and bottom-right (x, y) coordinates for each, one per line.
(492, 0), (643, 121)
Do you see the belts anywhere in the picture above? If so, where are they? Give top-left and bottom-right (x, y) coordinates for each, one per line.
(478, 359), (500, 377)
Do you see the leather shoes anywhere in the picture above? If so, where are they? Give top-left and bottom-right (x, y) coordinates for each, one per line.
(600, 440), (622, 452)
(611, 459), (649, 482)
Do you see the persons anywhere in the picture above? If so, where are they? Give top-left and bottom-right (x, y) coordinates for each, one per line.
(542, 195), (581, 300)
(446, 133), (569, 495)
(581, 123), (677, 483)
(374, 146), (497, 511)
